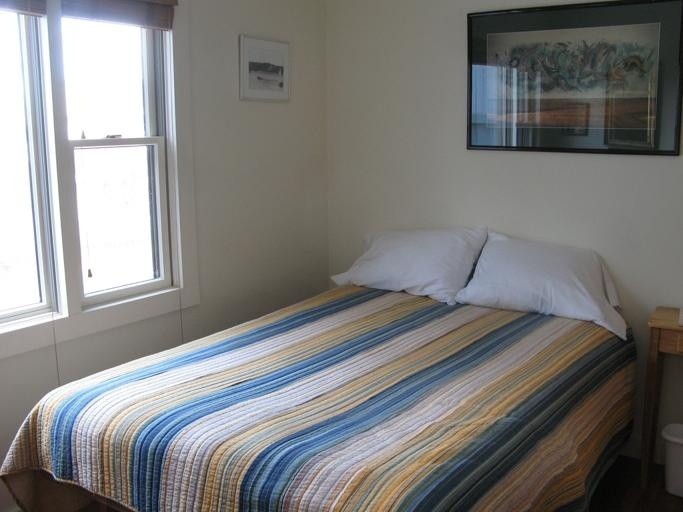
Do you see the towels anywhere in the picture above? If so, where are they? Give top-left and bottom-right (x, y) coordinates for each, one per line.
(662, 423), (683, 498)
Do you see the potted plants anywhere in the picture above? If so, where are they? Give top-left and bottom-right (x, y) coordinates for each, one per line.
(239, 34), (291, 101)
(466, 0), (683, 156)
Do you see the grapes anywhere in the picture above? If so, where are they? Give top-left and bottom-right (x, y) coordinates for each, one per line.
(635, 306), (683, 493)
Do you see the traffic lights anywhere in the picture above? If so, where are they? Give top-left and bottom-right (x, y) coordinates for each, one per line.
(330, 224), (491, 305)
(454, 231), (627, 342)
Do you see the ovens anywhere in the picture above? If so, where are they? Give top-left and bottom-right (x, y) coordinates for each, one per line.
(0, 283), (636, 511)
